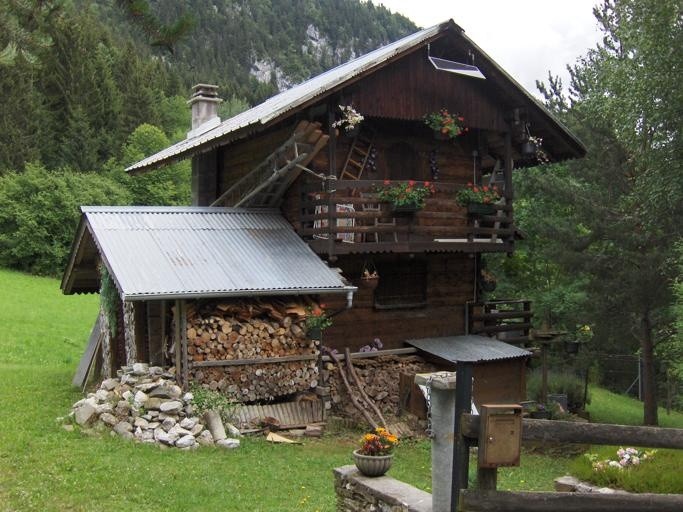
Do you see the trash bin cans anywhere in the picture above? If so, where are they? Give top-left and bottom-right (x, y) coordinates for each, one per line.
(546, 393), (568, 411)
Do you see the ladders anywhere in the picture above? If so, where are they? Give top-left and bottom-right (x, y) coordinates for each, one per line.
(237, 120), (330, 208)
(209, 129), (308, 208)
(474, 147), (515, 243)
(339, 125), (377, 196)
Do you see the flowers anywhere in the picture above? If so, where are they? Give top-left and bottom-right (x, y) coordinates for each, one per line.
(453, 178), (503, 204)
(379, 174), (433, 209)
(355, 429), (395, 454)
(433, 129), (447, 141)
(304, 302), (332, 333)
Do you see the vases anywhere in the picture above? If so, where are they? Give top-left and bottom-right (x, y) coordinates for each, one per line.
(352, 448), (392, 475)
(392, 200), (416, 214)
(465, 201), (495, 214)
(424, 106), (469, 136)
